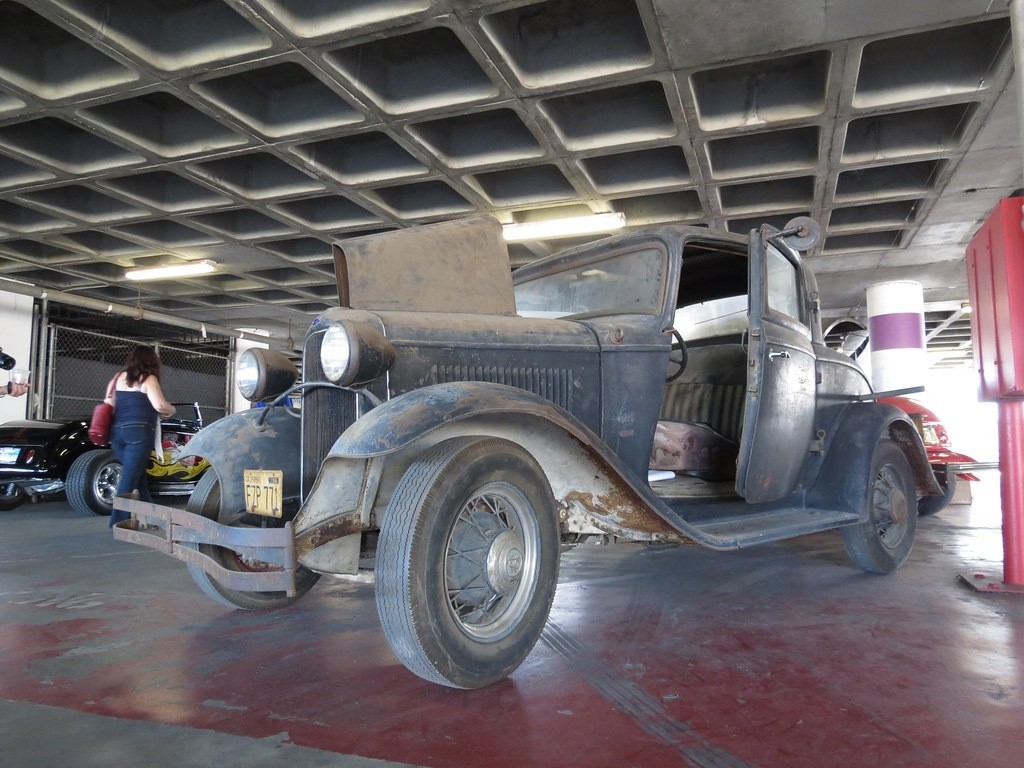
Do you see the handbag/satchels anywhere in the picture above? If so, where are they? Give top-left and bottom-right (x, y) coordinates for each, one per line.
(89, 371), (127, 446)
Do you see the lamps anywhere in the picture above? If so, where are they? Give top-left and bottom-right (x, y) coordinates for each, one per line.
(124, 259), (218, 281)
(502, 212), (628, 244)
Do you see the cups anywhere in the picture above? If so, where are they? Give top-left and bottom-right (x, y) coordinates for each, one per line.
(9, 369), (31, 384)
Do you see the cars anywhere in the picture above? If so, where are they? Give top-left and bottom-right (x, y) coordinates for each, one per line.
(875, 395), (979, 516)
(109, 213), (950, 693)
(0, 400), (213, 518)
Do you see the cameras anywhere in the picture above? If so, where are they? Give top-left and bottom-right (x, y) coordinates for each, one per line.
(0, 348), (16, 371)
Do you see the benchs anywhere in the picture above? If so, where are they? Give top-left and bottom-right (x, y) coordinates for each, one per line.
(648, 333), (748, 484)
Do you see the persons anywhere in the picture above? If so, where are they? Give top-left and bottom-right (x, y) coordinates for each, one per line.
(106, 346), (176, 528)
(0, 382), (30, 397)
